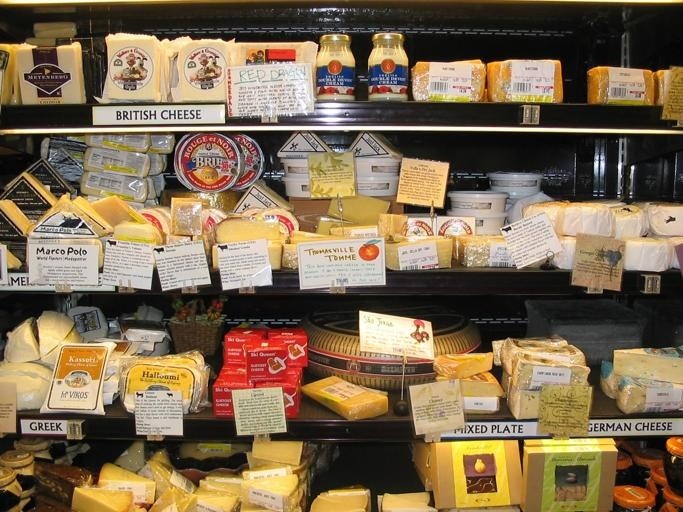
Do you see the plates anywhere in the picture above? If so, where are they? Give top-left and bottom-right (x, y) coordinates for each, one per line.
(165, 464), (250, 477)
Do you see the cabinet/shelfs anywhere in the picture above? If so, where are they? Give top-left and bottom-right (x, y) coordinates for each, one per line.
(0, 117), (683, 443)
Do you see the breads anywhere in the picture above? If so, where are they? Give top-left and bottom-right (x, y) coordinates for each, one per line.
(0, 21), (683, 512)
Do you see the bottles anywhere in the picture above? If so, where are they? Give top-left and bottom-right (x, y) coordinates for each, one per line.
(315, 33), (357, 103)
(0, 437), (54, 511)
(367, 32), (410, 103)
(663, 435), (683, 495)
